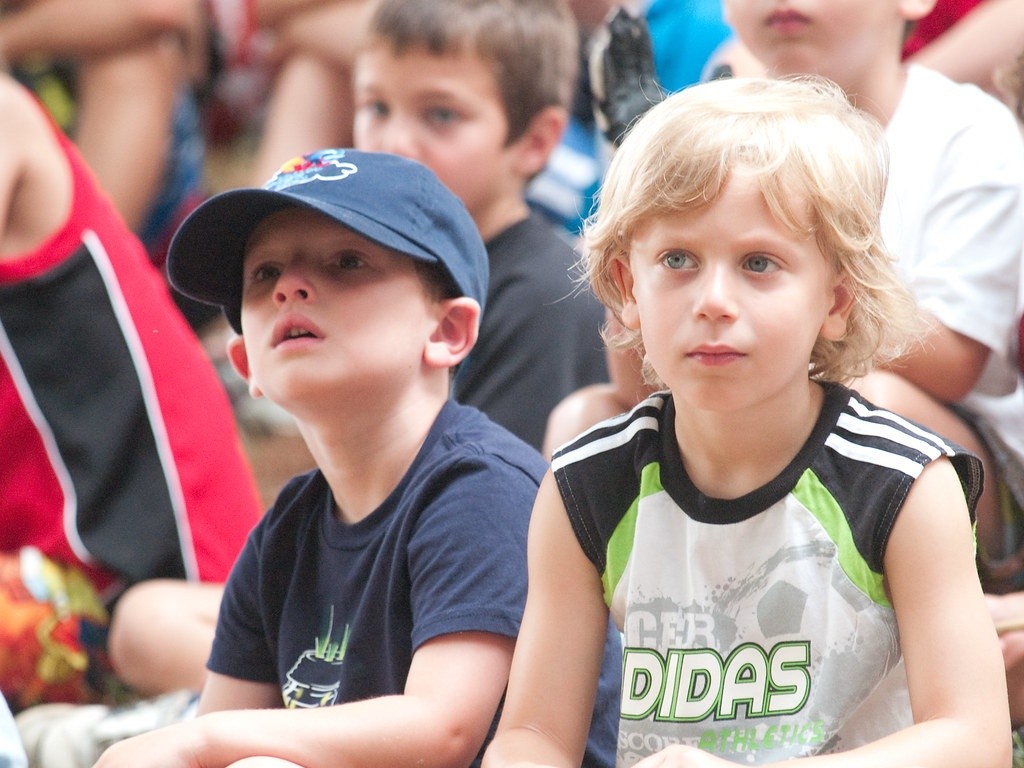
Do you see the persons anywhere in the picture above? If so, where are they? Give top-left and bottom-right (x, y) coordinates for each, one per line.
(1, 1), (1023, 768)
(95, 145), (624, 767)
(477, 76), (1014, 768)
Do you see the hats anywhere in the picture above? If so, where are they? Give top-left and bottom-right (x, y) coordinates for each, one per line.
(166, 147), (492, 327)
